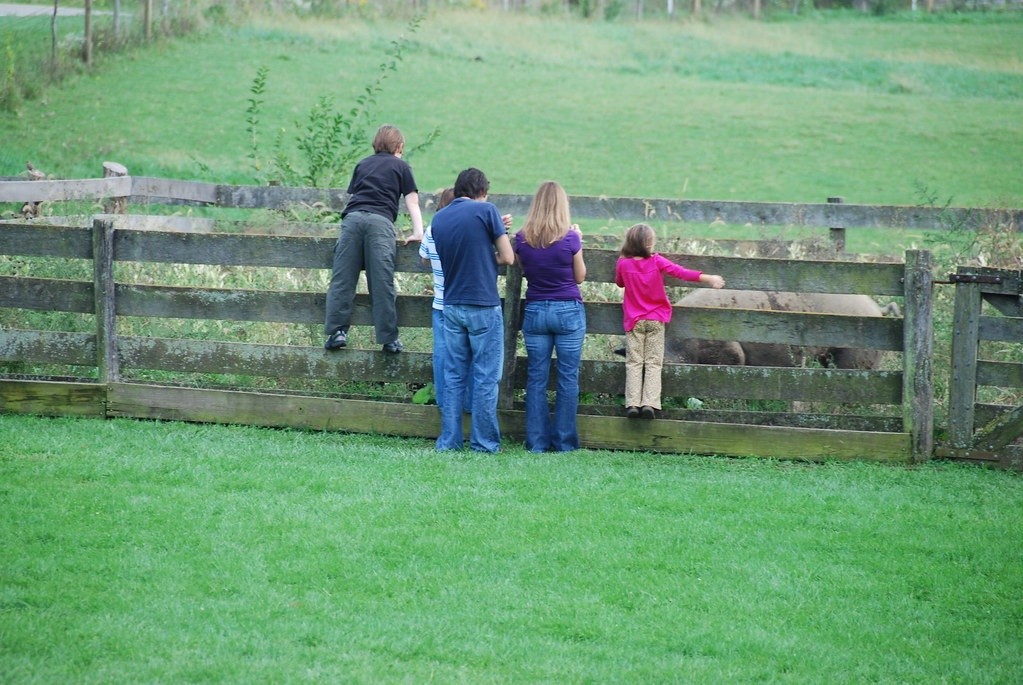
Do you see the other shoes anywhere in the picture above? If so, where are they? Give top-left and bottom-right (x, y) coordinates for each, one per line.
(627, 406), (640, 418)
(640, 406), (655, 419)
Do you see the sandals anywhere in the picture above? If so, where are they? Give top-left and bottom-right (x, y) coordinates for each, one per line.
(384, 341), (403, 353)
(324, 331), (347, 350)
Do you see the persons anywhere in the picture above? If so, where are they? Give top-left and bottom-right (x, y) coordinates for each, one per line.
(514, 179), (588, 452)
(417, 166), (514, 453)
(614, 222), (727, 418)
(323, 123), (423, 354)
